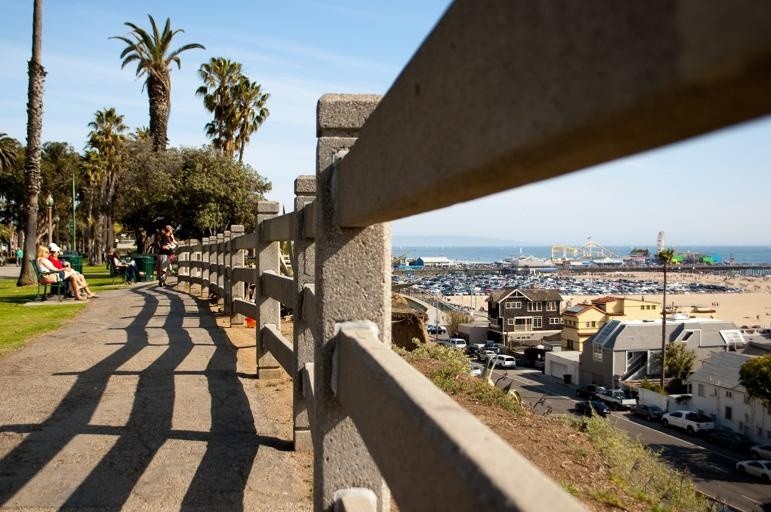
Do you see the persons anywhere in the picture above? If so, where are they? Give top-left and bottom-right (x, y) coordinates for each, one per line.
(36, 242), (99, 300)
(155, 225), (178, 287)
(113, 251), (147, 285)
(15, 246), (23, 267)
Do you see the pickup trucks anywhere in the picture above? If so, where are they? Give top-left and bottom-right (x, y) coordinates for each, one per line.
(661, 409), (716, 436)
(596, 389), (638, 411)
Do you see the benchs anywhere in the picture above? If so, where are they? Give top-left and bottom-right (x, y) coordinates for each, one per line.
(28, 258), (70, 301)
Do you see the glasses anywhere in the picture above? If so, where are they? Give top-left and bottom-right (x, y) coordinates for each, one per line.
(50, 251), (59, 253)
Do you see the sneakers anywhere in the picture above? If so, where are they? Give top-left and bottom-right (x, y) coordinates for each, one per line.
(74, 293), (100, 300)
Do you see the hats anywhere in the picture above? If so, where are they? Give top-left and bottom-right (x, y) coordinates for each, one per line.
(48, 243), (62, 251)
(165, 224), (174, 232)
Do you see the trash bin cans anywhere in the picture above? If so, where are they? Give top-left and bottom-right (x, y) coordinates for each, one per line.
(134, 256), (153, 282)
(50, 255), (83, 295)
(563, 375), (571, 384)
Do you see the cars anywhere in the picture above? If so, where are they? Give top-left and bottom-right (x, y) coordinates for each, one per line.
(425, 324), (447, 334)
(391, 274), (742, 296)
(736, 443), (771, 481)
(575, 383), (611, 417)
(437, 338), (517, 369)
(630, 403), (664, 421)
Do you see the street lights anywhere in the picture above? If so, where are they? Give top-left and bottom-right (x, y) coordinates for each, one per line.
(45, 193), (60, 247)
